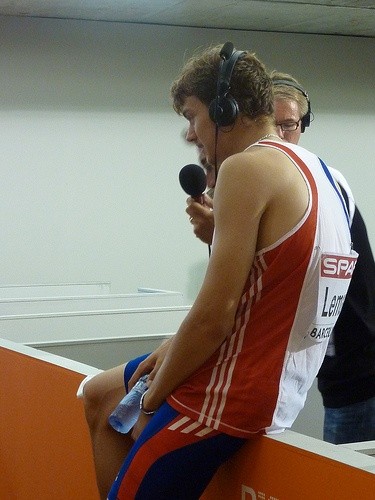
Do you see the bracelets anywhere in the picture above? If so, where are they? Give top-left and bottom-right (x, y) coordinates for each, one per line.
(139, 389), (155, 416)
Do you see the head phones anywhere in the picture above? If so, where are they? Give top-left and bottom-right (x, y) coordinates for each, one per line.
(271, 79), (311, 133)
(209, 48), (246, 126)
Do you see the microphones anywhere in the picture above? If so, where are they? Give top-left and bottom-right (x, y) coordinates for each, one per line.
(213, 41), (234, 121)
(179, 164), (206, 206)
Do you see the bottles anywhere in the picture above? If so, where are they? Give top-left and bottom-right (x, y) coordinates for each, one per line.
(108, 374), (154, 434)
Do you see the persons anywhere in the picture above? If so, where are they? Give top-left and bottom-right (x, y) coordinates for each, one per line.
(185, 71), (375, 444)
(83, 43), (352, 500)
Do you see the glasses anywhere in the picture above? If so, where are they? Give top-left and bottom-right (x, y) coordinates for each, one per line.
(275, 117), (301, 131)
